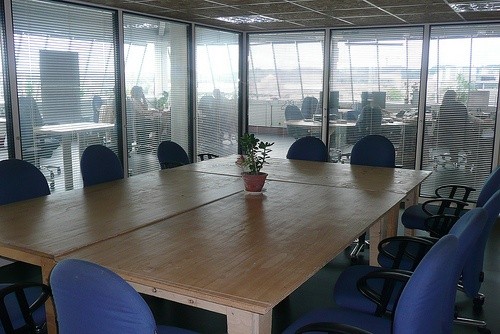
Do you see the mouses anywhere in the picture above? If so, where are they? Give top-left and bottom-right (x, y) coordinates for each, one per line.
(389, 120), (393, 123)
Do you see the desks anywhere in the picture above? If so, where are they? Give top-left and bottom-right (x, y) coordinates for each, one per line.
(54, 181), (407, 334)
(338, 109), (354, 120)
(172, 154), (432, 240)
(285, 120), (410, 151)
(34, 122), (115, 191)
(0, 153), (432, 334)
(0, 170), (269, 334)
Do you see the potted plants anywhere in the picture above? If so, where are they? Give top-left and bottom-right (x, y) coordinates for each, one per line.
(235, 133), (275, 195)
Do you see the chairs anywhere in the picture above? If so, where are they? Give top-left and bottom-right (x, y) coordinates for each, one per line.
(281, 136), (500, 334)
(0, 141), (198, 334)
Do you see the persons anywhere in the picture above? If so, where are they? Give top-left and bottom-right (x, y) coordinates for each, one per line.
(442, 90), (484, 169)
(131, 86), (158, 116)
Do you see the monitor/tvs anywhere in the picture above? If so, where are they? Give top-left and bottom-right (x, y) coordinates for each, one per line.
(320, 91), (339, 108)
(362, 92), (386, 109)
(467, 91), (490, 108)
